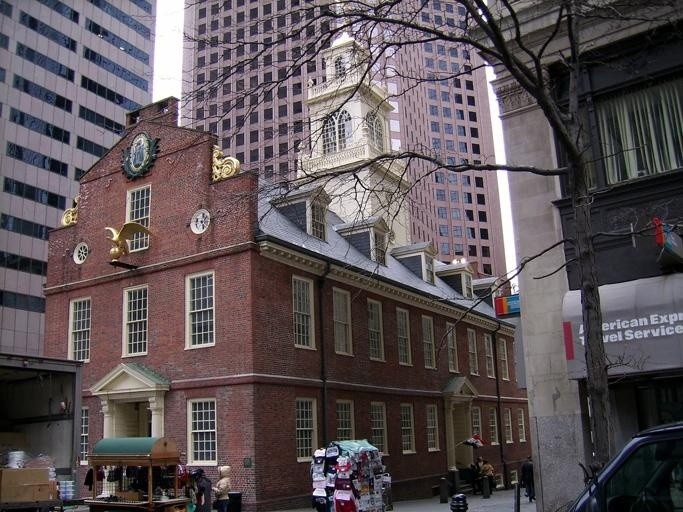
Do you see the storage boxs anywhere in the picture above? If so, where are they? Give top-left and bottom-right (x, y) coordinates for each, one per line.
(0, 466), (49, 503)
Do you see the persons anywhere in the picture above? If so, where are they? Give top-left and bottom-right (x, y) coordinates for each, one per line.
(212, 465), (231, 512)
(476, 455), (536, 503)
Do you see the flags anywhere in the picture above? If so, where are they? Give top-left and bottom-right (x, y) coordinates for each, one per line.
(463, 435), (488, 449)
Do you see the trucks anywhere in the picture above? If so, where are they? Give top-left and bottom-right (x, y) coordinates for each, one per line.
(0, 351), (91, 510)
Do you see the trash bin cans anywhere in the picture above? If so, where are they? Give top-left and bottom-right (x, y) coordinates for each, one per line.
(227, 491), (242, 512)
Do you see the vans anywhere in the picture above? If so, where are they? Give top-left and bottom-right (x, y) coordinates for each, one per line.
(553, 420), (683, 512)
